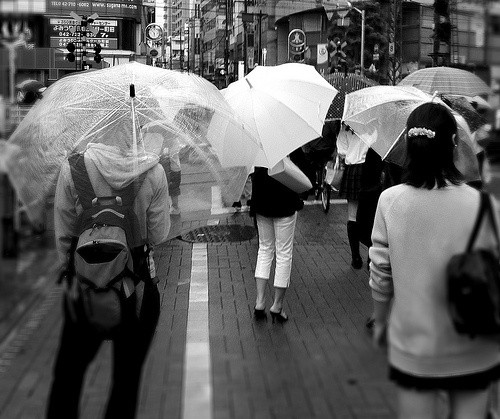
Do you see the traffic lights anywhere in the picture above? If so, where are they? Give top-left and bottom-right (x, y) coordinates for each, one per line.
(216, 67), (227, 90)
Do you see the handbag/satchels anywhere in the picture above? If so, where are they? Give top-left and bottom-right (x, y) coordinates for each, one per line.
(268, 156), (313, 193)
(445, 193), (500, 343)
(325, 155), (345, 193)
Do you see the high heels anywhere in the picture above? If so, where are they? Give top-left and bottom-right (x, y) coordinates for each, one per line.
(269, 308), (288, 325)
(254, 306), (266, 321)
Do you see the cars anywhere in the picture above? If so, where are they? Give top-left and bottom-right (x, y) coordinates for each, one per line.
(17, 79), (46, 106)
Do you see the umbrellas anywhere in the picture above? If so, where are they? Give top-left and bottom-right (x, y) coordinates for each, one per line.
(207, 62), (339, 169)
(396, 66), (495, 96)
(0, 62), (263, 244)
(342, 85), (482, 185)
(317, 72), (384, 121)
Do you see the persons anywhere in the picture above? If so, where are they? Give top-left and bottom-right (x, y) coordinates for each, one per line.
(13, 78), (47, 107)
(45, 102), (170, 419)
(369, 102), (500, 419)
(472, 130), (500, 199)
(160, 125), (181, 217)
(249, 147), (315, 324)
(336, 147), (386, 270)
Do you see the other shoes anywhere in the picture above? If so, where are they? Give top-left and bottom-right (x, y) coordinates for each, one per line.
(170, 205), (181, 215)
(352, 258), (362, 270)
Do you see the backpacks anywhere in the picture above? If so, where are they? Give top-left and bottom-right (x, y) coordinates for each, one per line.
(67, 151), (144, 336)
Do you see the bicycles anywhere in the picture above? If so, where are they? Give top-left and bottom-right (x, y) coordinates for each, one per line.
(313, 163), (331, 214)
